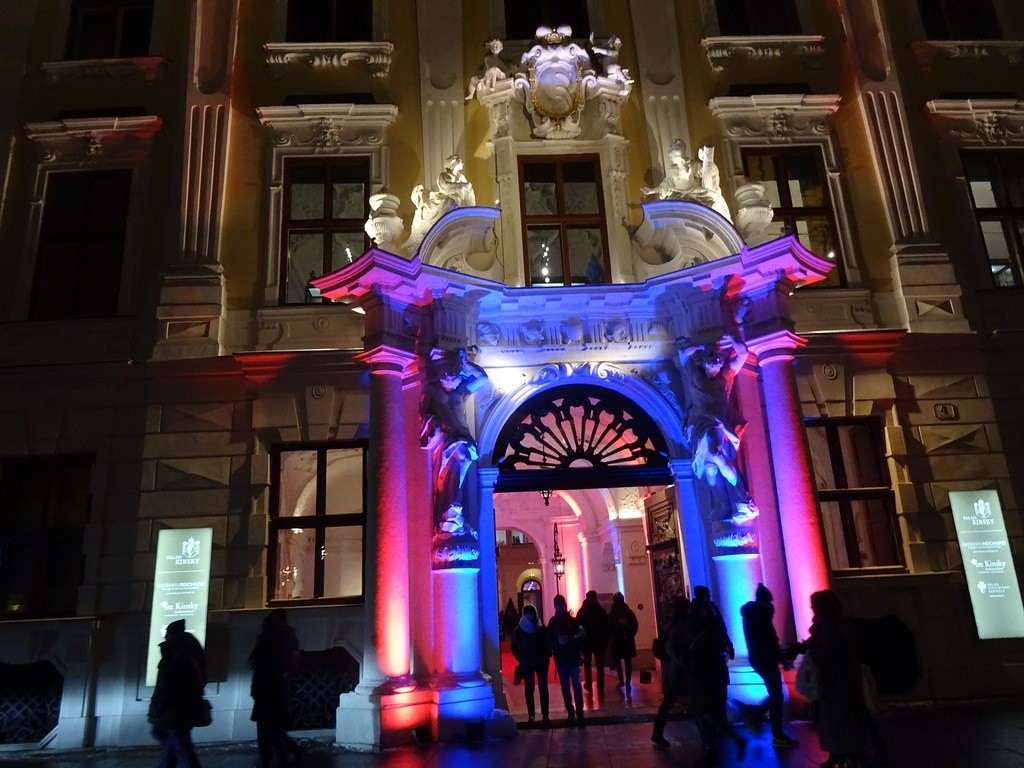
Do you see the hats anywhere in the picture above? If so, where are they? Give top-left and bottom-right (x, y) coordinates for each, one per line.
(167, 619), (185, 633)
(756, 582), (774, 601)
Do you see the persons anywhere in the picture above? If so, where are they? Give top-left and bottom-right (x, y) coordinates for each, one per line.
(608, 593), (638, 690)
(639, 139), (733, 225)
(548, 596), (586, 724)
(249, 608), (306, 768)
(651, 584), (891, 768)
(464, 38), (511, 100)
(147, 619), (208, 768)
(510, 605), (551, 724)
(591, 35), (634, 95)
(576, 591), (608, 697)
(400, 154), (475, 259)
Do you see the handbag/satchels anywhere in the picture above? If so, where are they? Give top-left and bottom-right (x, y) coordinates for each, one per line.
(193, 700), (212, 727)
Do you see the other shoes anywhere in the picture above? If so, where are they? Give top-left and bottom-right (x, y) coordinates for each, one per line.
(292, 746), (307, 765)
(694, 753), (720, 768)
(772, 736), (800, 752)
(528, 715), (536, 724)
(543, 715), (551, 722)
(583, 683), (592, 693)
(745, 715), (763, 733)
(568, 714), (576, 726)
(651, 734), (670, 747)
(736, 738), (746, 760)
(597, 691), (604, 699)
(577, 714), (585, 726)
(616, 681), (624, 689)
(626, 686), (631, 691)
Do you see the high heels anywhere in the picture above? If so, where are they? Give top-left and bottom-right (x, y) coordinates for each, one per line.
(819, 752), (845, 768)
